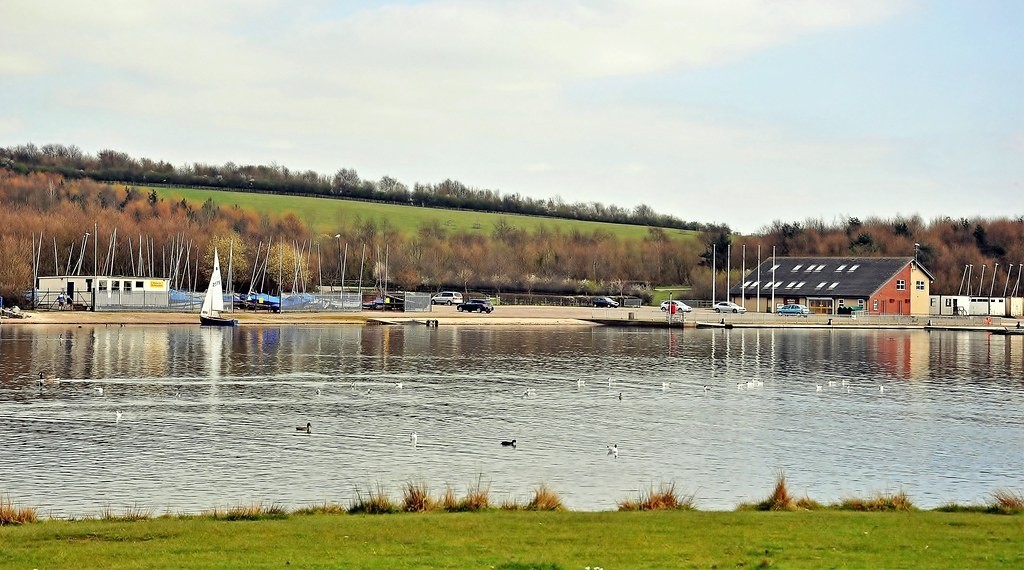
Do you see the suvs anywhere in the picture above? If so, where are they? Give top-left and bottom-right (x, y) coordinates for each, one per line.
(457, 299), (494, 314)
(592, 297), (619, 308)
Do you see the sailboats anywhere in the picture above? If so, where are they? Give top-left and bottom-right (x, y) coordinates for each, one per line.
(169, 232), (200, 301)
(317, 243), (390, 309)
(200, 249), (238, 326)
(223, 236), (316, 309)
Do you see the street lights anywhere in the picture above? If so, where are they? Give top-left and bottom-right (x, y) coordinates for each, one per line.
(335, 234), (342, 286)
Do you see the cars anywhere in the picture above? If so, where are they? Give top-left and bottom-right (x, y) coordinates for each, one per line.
(713, 302), (747, 314)
(776, 304), (809, 318)
(660, 300), (692, 313)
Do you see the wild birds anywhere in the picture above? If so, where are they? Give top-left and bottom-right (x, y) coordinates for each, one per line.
(36, 322), (886, 461)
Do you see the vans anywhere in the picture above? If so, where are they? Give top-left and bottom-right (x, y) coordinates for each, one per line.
(431, 292), (464, 306)
